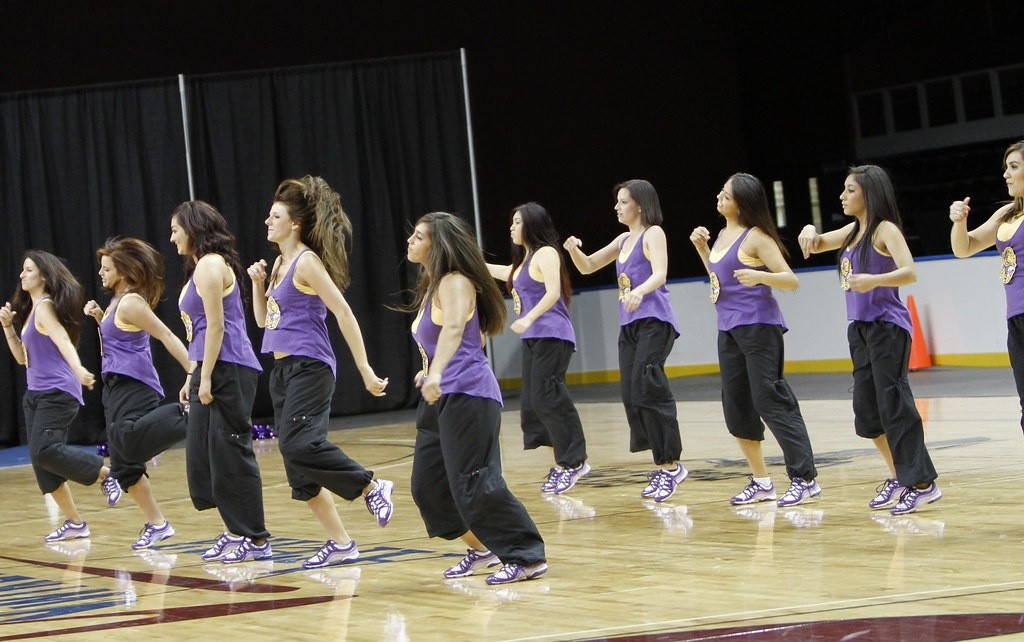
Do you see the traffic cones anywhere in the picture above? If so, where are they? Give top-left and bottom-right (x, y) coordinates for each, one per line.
(907, 294), (932, 372)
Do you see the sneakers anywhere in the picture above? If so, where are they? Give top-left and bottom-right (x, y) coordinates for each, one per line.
(653, 463), (688, 502)
(364, 479), (394, 528)
(131, 519), (175, 550)
(443, 548), (502, 578)
(554, 462), (598, 494)
(200, 531), (245, 562)
(869, 479), (907, 510)
(729, 476), (776, 504)
(777, 477), (822, 507)
(220, 537), (272, 563)
(889, 481), (942, 515)
(484, 561), (548, 585)
(302, 539), (360, 569)
(539, 466), (565, 492)
(640, 469), (662, 498)
(45, 519), (91, 542)
(100, 466), (122, 507)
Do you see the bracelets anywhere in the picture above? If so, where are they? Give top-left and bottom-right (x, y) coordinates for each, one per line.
(2, 324), (12, 327)
(186, 373), (192, 375)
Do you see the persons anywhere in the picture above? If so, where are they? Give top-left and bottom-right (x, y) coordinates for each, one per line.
(562, 179), (689, 501)
(247, 176), (394, 569)
(797, 165), (941, 518)
(84, 238), (191, 550)
(689, 173), (821, 508)
(950, 138), (1024, 435)
(170, 201), (272, 563)
(383, 212), (548, 585)
(483, 202), (591, 495)
(0, 251), (122, 543)
